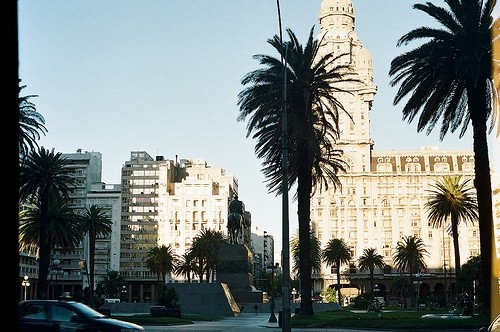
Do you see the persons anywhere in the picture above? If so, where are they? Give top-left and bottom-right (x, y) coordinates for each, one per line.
(255, 304), (258, 314)
(226, 195), (247, 228)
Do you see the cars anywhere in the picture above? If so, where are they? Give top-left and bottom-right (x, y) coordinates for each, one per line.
(19, 300), (146, 332)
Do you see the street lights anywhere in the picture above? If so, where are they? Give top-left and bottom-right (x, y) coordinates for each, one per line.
(265, 260), (280, 323)
(50, 259), (63, 300)
(22, 275), (30, 300)
(413, 274), (422, 311)
